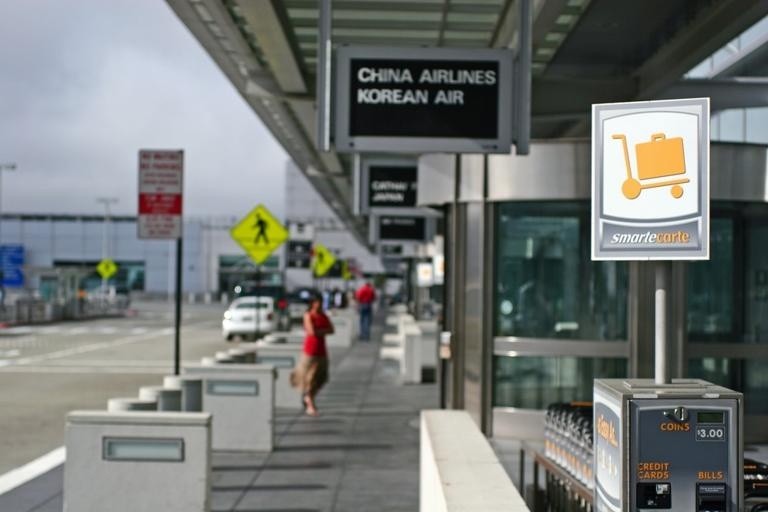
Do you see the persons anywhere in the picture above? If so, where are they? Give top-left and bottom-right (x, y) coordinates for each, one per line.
(352, 280), (376, 340)
(291, 294), (334, 416)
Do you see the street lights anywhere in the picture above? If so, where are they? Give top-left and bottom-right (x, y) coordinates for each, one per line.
(0, 164), (16, 191)
(95, 198), (119, 287)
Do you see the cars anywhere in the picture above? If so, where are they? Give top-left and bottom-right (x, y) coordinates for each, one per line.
(85, 285), (130, 310)
(222, 280), (311, 340)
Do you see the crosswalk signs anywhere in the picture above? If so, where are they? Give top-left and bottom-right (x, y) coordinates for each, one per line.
(230, 204), (288, 266)
